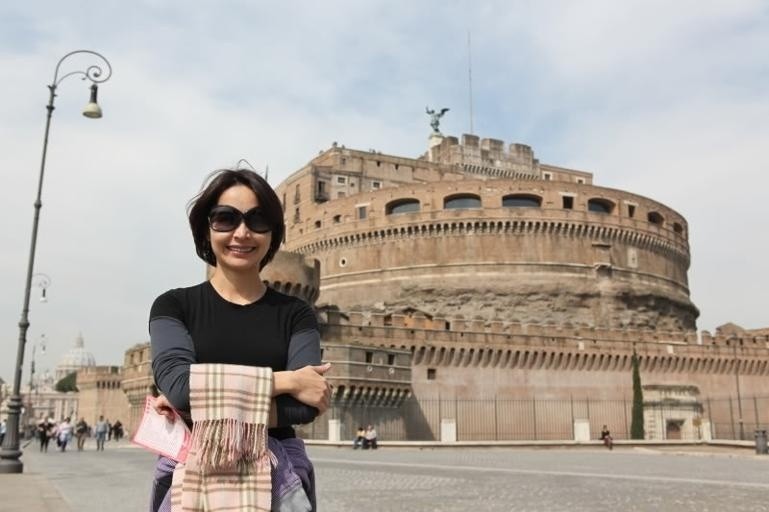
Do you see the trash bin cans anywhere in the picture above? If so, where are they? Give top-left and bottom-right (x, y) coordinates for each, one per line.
(754, 430), (767, 454)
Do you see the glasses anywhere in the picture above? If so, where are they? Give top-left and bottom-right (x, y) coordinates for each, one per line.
(205, 204), (276, 235)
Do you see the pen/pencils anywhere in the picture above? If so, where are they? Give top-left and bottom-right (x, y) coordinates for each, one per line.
(150, 385), (159, 397)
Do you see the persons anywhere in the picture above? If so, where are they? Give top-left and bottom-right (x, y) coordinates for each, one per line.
(353, 427), (366, 449)
(19, 422), (25, 435)
(35, 414), (125, 453)
(147, 168), (334, 512)
(0, 419), (7, 445)
(601, 425), (613, 450)
(364, 424), (377, 449)
